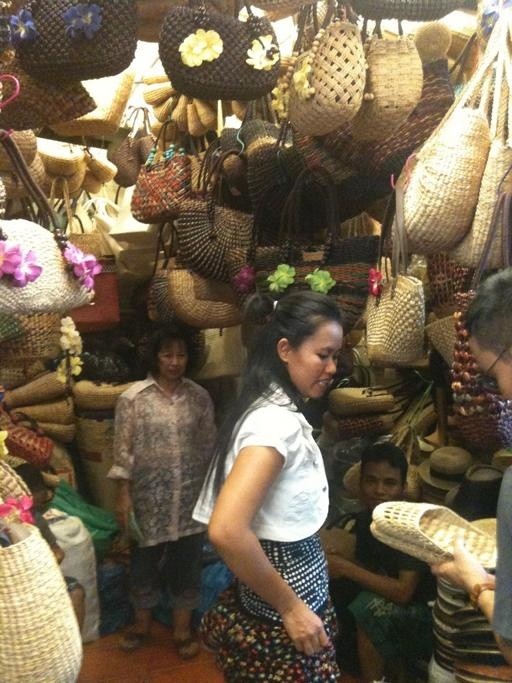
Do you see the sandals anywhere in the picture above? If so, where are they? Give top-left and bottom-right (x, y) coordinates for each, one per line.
(117, 626), (144, 653)
(173, 633), (198, 659)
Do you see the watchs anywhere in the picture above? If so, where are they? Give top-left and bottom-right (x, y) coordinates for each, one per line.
(469, 583), (496, 613)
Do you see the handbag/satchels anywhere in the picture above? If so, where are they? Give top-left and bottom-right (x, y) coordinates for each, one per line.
(9, 0), (139, 79)
(143, 73), (217, 136)
(142, 221), (178, 321)
(179, 150), (255, 282)
(0, 56), (97, 131)
(45, 74), (133, 137)
(322, 402), (408, 442)
(353, 15), (423, 154)
(0, 497), (83, 683)
(129, 119), (189, 223)
(427, 317), (456, 366)
(3, 371), (67, 407)
(158, 0), (281, 102)
(327, 380), (410, 415)
(219, 95), (290, 200)
(189, 135), (216, 194)
(170, 269), (245, 326)
(365, 186), (425, 367)
(254, 166), (379, 331)
(449, 32), (512, 271)
(318, 60), (450, 178)
(451, 191), (512, 451)
(287, 1), (367, 138)
(388, 4), (504, 255)
(18, 421), (76, 444)
(10, 397), (74, 424)
(49, 179), (122, 331)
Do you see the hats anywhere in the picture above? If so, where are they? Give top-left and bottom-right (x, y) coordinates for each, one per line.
(418, 445), (472, 491)
(443, 465), (503, 521)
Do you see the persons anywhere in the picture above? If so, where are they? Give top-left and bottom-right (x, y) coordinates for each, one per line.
(190, 292), (343, 683)
(430, 266), (512, 683)
(105, 327), (217, 661)
(327, 442), (432, 683)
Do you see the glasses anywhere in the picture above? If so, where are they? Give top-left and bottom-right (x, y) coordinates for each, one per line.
(477, 347), (507, 394)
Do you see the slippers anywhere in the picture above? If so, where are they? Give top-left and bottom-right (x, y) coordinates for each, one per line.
(371, 499), (498, 572)
(368, 521), (453, 566)
